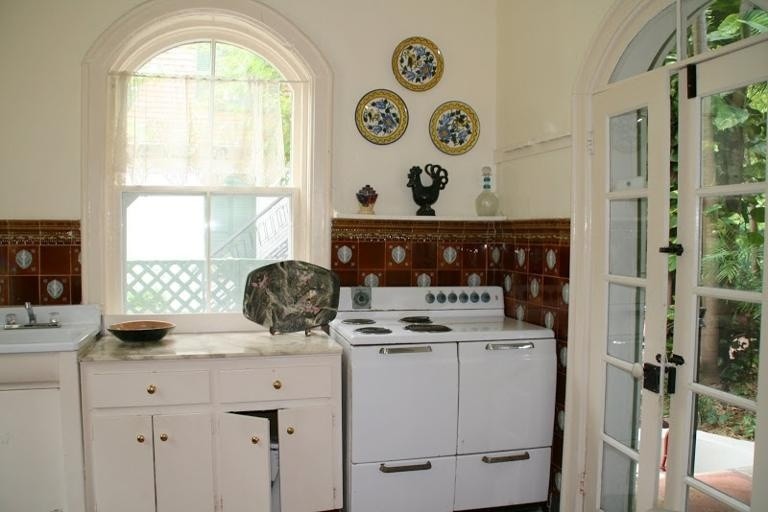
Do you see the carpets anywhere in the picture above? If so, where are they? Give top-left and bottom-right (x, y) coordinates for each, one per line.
(632, 470), (752, 511)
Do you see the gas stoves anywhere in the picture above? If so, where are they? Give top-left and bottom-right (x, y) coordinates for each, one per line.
(339, 314), (454, 339)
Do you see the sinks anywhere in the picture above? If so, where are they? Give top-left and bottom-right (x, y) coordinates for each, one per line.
(0, 323), (99, 353)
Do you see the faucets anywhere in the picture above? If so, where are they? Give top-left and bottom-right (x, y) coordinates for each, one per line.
(24, 301), (36, 325)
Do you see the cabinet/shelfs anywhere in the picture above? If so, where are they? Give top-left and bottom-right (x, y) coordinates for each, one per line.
(214, 352), (345, 512)
(81, 353), (214, 512)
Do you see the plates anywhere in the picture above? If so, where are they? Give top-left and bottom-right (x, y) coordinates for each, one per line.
(352, 36), (482, 156)
(105, 319), (176, 343)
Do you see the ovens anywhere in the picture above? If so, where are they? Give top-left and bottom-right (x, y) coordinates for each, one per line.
(344, 337), (557, 511)
